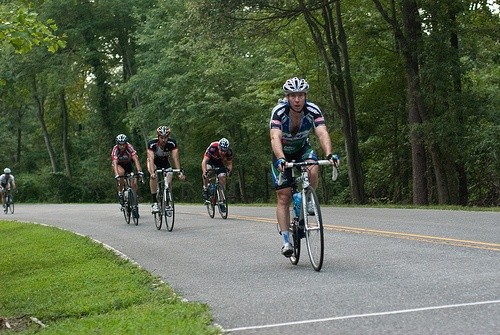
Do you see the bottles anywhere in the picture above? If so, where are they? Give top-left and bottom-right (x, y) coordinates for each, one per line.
(124, 189), (128, 201)
(293, 192), (302, 216)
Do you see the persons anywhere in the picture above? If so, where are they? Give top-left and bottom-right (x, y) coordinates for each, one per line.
(269, 77), (339, 258)
(0, 168), (16, 211)
(146, 126), (185, 213)
(202, 139), (234, 205)
(112, 134), (144, 219)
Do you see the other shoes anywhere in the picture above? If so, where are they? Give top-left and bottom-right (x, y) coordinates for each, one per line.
(3, 208), (6, 211)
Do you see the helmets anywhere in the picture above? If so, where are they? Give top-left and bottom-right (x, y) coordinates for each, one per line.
(157, 126), (171, 137)
(4, 168), (11, 173)
(116, 134), (128, 144)
(218, 138), (230, 150)
(283, 77), (309, 96)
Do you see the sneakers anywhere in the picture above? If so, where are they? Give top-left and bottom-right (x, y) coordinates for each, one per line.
(220, 205), (226, 212)
(132, 207), (139, 217)
(308, 201), (315, 215)
(119, 195), (124, 204)
(165, 206), (172, 217)
(281, 242), (293, 255)
(153, 202), (161, 212)
(202, 190), (208, 199)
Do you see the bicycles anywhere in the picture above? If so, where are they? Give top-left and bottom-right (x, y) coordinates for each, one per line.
(2, 188), (19, 214)
(282, 157), (338, 270)
(115, 174), (147, 226)
(151, 168), (185, 232)
(203, 167), (229, 219)
